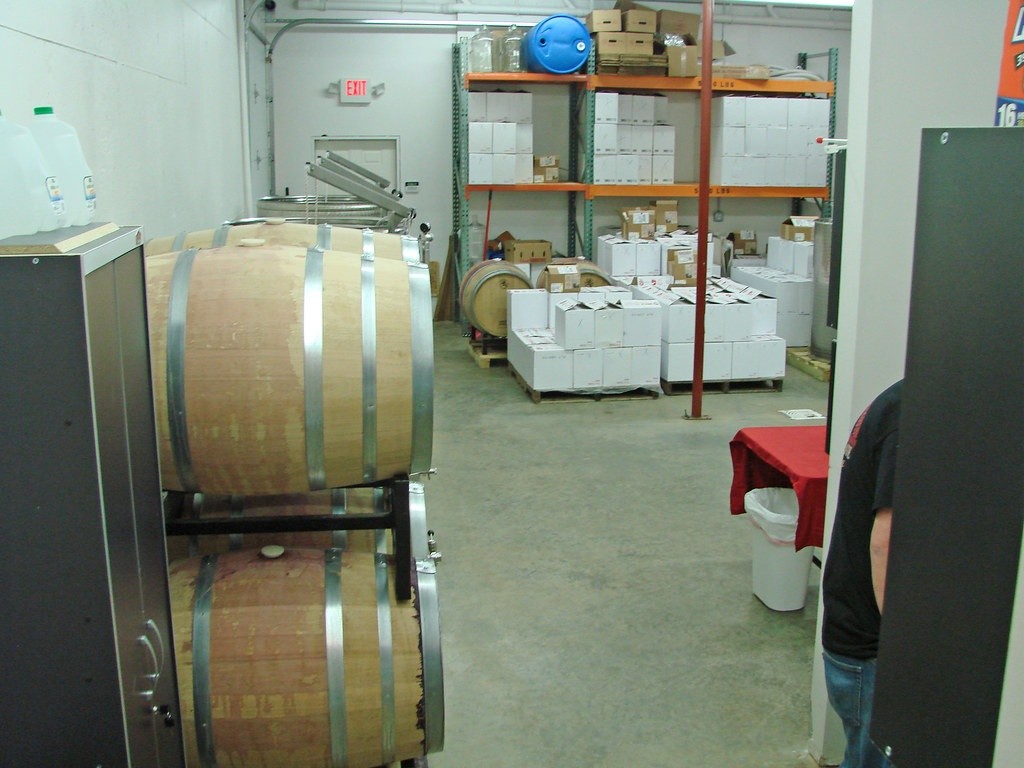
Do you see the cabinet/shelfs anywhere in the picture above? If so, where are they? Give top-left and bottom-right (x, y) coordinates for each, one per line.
(453, 45), (841, 335)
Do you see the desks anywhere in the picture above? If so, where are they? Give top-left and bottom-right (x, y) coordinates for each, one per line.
(729, 426), (829, 569)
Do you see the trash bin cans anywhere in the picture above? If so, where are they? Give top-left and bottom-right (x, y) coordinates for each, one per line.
(742, 486), (814, 612)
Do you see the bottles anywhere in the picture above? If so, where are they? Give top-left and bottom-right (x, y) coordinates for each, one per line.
(467, 22), (529, 74)
(468, 214), (487, 264)
(0, 105), (97, 236)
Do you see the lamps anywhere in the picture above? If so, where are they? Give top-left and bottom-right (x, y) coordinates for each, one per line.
(327, 77), (388, 104)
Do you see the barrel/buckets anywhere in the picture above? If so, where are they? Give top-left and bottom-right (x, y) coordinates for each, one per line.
(141, 218), (437, 501)
(522, 13), (591, 75)
(162, 471), (446, 768)
(458, 259), (535, 339)
(536, 257), (614, 289)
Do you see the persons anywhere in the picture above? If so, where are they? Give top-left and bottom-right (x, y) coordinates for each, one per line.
(822, 378), (903, 768)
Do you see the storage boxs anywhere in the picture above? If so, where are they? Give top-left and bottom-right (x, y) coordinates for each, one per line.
(587, 0), (772, 80)
(695, 98), (832, 186)
(495, 198), (821, 393)
(588, 93), (682, 186)
(468, 88), (561, 183)
